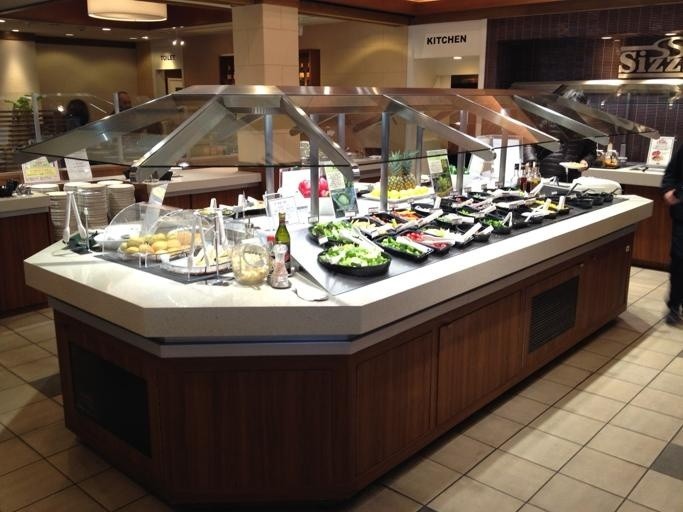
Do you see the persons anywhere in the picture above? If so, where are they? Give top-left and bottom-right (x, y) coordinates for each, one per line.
(110, 91), (134, 115)
(662, 136), (683, 326)
(524, 89), (597, 181)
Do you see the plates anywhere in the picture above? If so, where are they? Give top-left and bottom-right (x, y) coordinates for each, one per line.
(559, 162), (583, 171)
(63, 179), (136, 226)
(26, 182), (78, 241)
(306, 188), (618, 277)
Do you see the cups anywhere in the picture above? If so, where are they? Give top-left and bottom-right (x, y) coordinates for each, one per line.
(159, 167), (184, 182)
(0, 179), (19, 197)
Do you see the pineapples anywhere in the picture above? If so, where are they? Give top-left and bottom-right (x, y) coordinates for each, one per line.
(402, 173), (416, 190)
(387, 176), (404, 191)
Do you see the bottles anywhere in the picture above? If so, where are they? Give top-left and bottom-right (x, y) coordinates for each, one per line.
(223, 64), (234, 84)
(512, 159), (539, 192)
(299, 62), (310, 85)
(267, 213), (292, 290)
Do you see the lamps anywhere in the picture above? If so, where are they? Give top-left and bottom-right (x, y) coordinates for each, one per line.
(87, 0), (167, 22)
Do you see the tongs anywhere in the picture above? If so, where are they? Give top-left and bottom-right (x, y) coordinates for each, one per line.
(64, 192), (88, 243)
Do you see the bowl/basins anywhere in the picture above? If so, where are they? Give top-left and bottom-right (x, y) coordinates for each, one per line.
(93, 232), (131, 251)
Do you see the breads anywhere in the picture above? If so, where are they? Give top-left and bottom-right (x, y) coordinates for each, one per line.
(121, 230), (200, 256)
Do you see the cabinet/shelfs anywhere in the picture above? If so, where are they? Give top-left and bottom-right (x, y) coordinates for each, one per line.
(219, 49), (320, 86)
